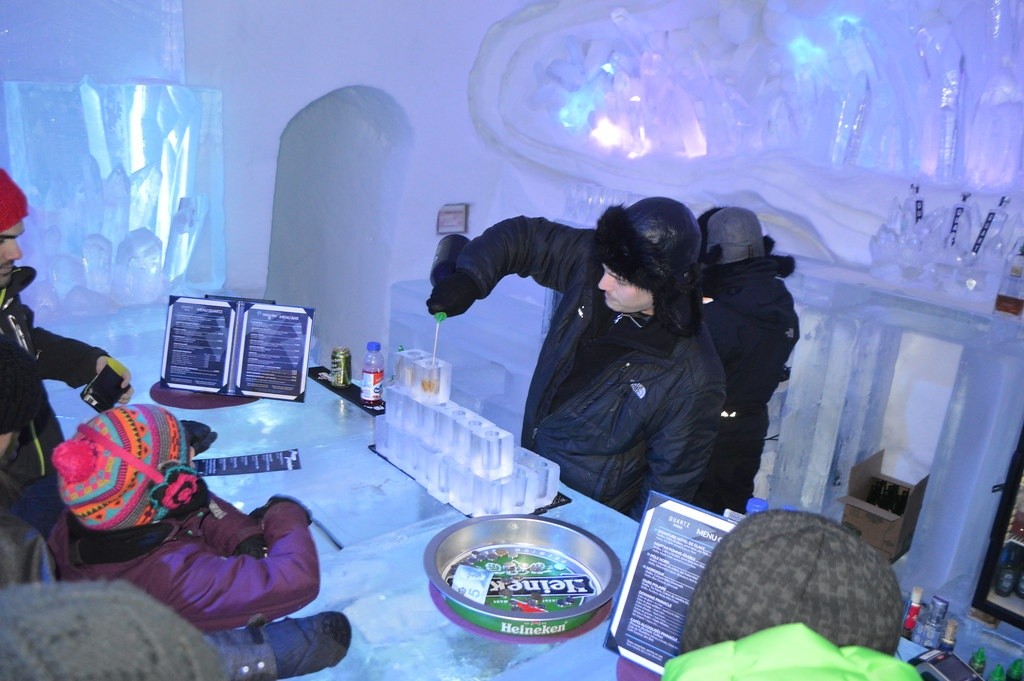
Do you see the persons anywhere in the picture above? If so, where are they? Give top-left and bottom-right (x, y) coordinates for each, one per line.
(46, 403), (322, 631)
(659, 507), (924, 681)
(0, 333), (353, 681)
(426, 197), (726, 522)
(698, 205), (800, 517)
(0, 167), (133, 528)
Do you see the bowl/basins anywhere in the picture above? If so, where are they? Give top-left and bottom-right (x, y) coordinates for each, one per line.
(424, 513), (623, 632)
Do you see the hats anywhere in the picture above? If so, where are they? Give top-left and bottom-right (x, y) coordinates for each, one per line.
(0, 169), (29, 238)
(678, 510), (906, 666)
(0, 333), (42, 434)
(697, 208), (775, 264)
(593, 197), (702, 336)
(52, 405), (211, 532)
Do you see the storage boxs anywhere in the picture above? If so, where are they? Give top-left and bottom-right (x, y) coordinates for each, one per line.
(835, 450), (930, 564)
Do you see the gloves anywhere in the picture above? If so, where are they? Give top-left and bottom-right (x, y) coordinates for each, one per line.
(179, 418), (217, 456)
(248, 494), (311, 525)
(425, 271), (478, 316)
(231, 535), (268, 563)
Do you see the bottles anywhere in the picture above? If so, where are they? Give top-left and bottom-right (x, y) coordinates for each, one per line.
(994, 546), (1024, 598)
(866, 480), (909, 515)
(995, 234), (1024, 315)
(360, 342), (384, 406)
(969, 647), (1024, 681)
(430, 234), (471, 324)
(901, 586), (957, 652)
(746, 497), (796, 519)
